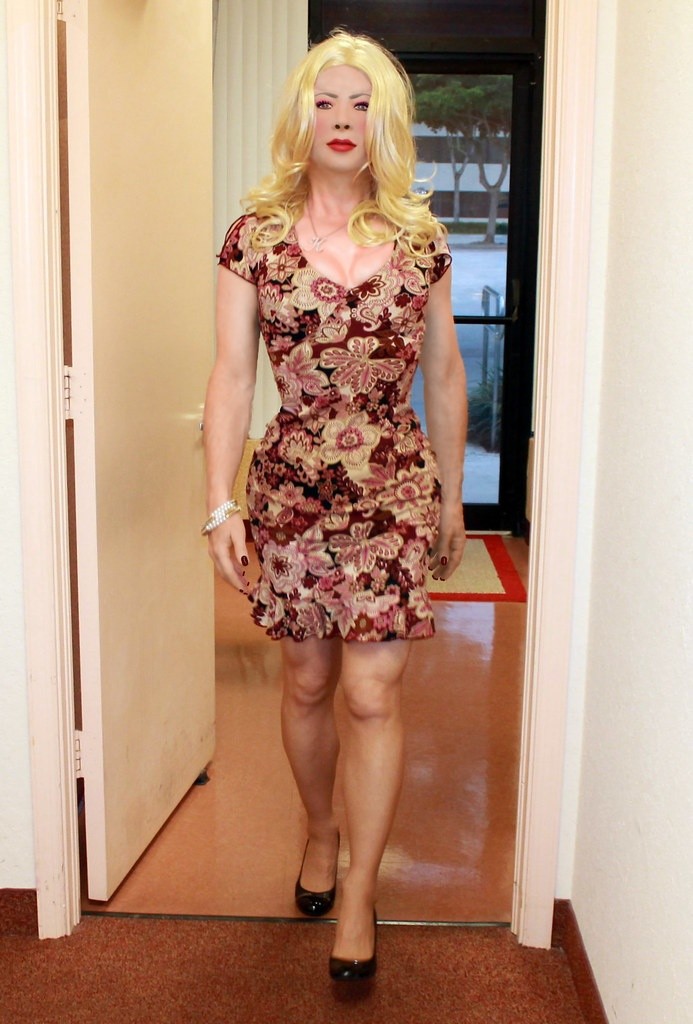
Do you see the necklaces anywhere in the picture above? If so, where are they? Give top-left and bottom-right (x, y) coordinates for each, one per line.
(305, 196), (348, 254)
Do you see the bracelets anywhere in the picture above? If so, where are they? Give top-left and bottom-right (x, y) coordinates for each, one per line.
(200, 500), (242, 536)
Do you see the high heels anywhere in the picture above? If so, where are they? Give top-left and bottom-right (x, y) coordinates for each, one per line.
(295, 826), (341, 917)
(327, 905), (376, 983)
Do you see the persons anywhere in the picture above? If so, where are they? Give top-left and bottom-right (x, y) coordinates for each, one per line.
(198, 33), (468, 981)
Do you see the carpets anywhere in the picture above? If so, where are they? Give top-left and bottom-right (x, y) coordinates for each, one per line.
(426, 533), (529, 605)
